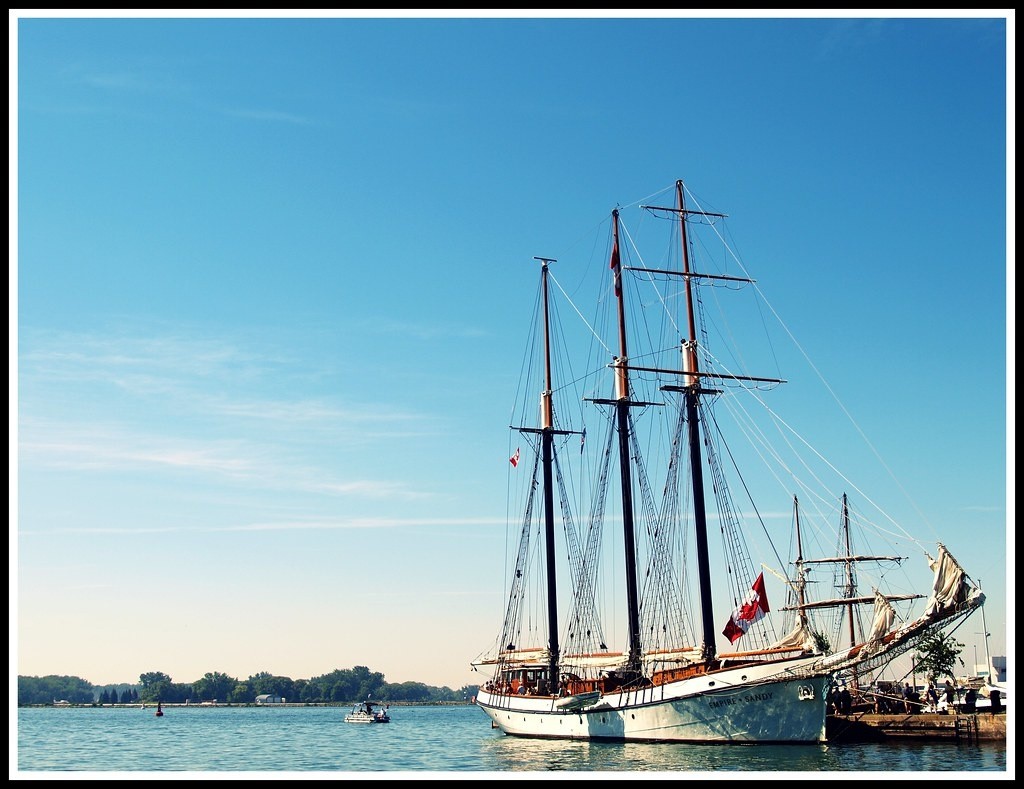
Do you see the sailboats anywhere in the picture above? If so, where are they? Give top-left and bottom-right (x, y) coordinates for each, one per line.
(465, 172), (993, 744)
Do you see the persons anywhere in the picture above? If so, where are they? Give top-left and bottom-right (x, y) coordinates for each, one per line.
(359, 703), (385, 717)
(875, 679), (955, 715)
(484, 678), (536, 695)
(832, 685), (852, 716)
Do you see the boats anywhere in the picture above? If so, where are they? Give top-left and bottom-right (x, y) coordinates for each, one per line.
(343, 708), (392, 724)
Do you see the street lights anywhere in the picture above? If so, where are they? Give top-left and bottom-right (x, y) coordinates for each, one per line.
(909, 652), (918, 694)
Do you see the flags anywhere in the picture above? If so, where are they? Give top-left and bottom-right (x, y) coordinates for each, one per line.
(510, 446), (520, 468)
(721, 570), (771, 646)
